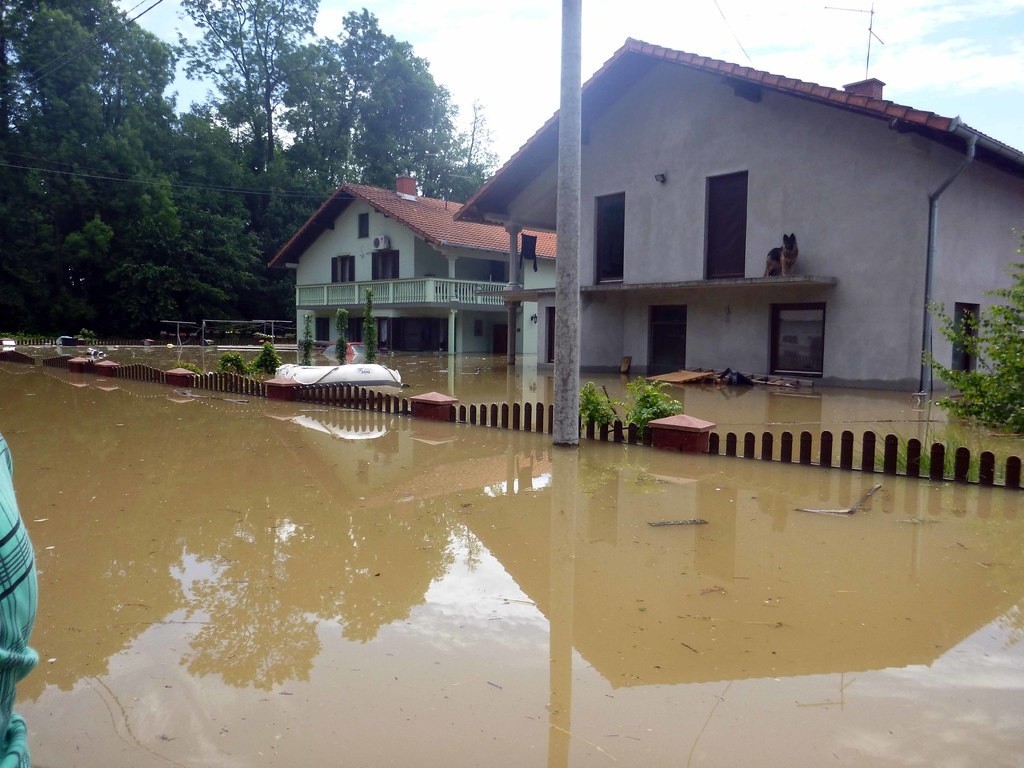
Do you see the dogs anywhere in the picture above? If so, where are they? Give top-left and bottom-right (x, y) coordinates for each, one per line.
(763, 232), (799, 277)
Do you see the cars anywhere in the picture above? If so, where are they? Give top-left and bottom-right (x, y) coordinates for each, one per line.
(321, 341), (380, 362)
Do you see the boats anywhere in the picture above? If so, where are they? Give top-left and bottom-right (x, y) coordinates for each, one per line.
(274, 363), (404, 401)
